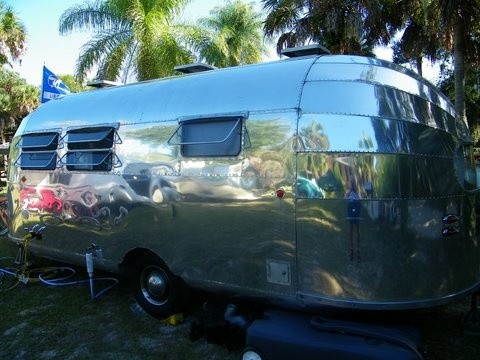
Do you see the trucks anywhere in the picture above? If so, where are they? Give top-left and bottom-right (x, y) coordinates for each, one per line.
(9, 42), (480, 321)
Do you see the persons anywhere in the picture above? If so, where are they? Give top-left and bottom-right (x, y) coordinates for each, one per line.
(346, 175), (361, 265)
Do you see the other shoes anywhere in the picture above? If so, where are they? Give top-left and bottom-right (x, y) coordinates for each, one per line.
(347, 249), (353, 260)
(355, 249), (363, 262)
